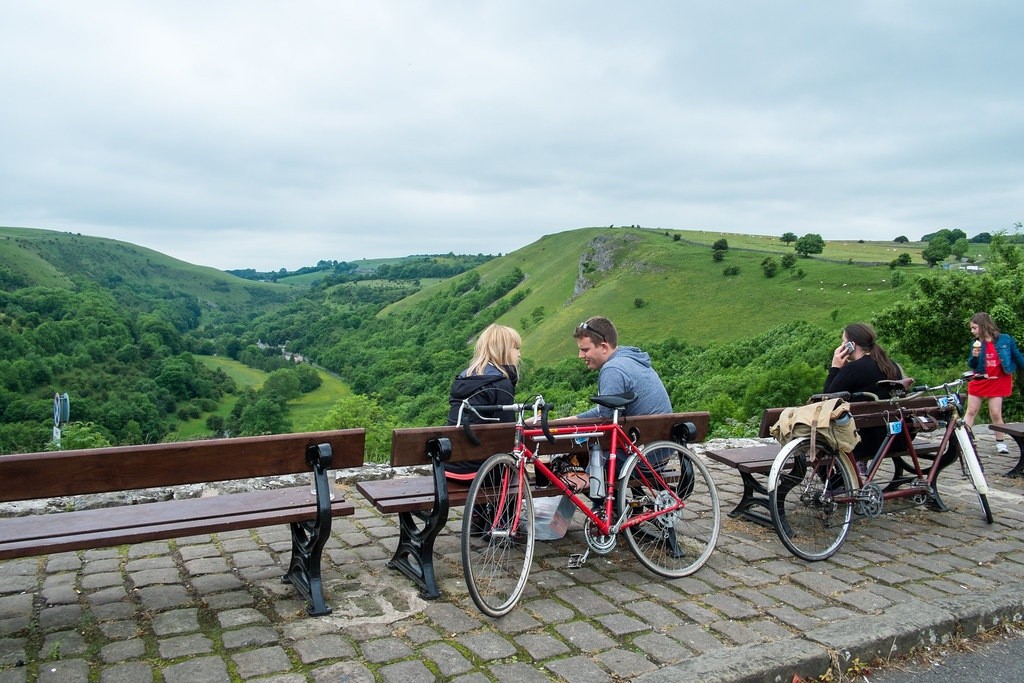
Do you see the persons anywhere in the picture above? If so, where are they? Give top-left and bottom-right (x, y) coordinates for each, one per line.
(823, 322), (918, 475)
(960, 313), (1024, 453)
(443, 323), (529, 548)
(541, 315), (678, 472)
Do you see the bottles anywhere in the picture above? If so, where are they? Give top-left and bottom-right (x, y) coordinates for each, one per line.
(835, 412), (850, 424)
(588, 444), (606, 498)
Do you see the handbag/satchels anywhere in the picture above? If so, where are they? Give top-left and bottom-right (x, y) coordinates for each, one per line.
(770, 397), (861, 453)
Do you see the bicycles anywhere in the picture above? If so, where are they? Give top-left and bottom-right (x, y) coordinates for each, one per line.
(450, 387), (723, 619)
(766, 370), (999, 563)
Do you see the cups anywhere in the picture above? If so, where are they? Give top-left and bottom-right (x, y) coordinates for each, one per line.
(536, 457), (551, 488)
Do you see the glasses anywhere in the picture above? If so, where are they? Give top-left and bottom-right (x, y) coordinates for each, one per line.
(579, 322), (607, 343)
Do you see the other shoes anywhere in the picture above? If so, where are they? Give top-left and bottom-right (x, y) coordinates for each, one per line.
(469, 514), (488, 537)
(483, 517), (524, 547)
(996, 443), (1009, 455)
(856, 465), (867, 475)
(820, 474), (847, 498)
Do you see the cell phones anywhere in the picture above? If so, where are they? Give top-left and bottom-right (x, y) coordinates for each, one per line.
(840, 342), (855, 358)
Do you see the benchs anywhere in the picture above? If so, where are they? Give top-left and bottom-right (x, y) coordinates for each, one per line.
(356, 411), (711, 600)
(989, 422), (1024, 480)
(0, 426), (367, 618)
(707, 393), (969, 538)
